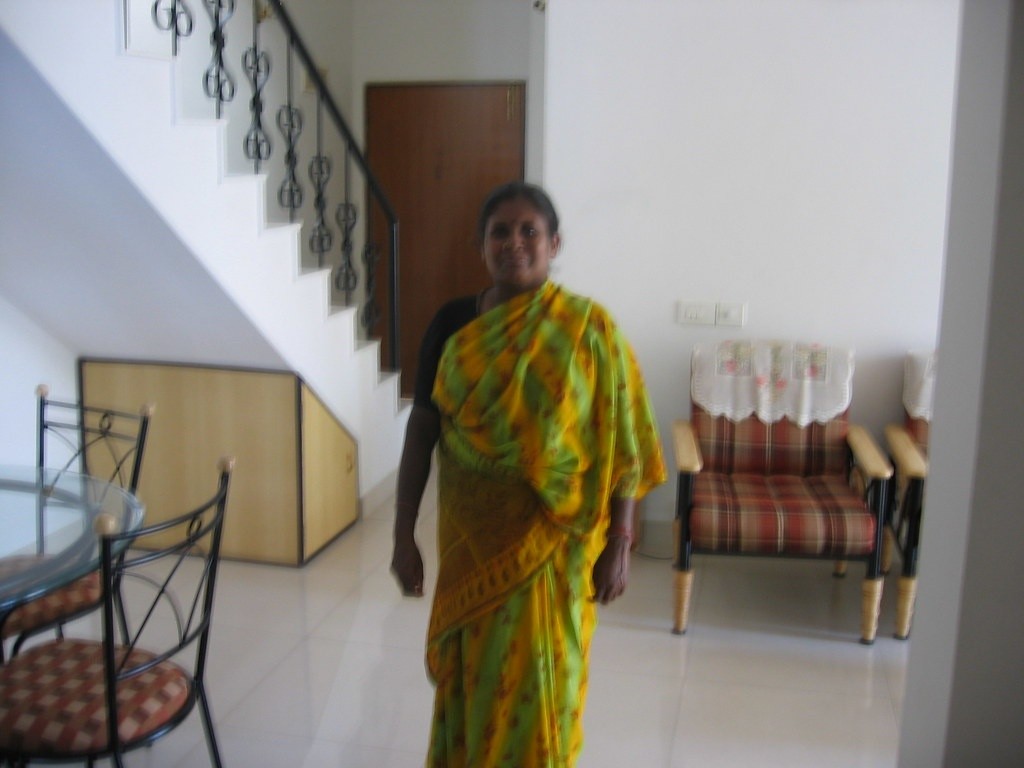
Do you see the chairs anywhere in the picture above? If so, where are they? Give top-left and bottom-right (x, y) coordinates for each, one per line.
(672, 338), (894, 647)
(0, 454), (233, 768)
(0, 385), (153, 673)
(878, 346), (935, 639)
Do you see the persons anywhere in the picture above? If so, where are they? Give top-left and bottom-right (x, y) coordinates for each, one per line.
(390, 181), (668, 768)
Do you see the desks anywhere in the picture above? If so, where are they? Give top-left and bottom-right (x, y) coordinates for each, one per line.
(0, 461), (145, 663)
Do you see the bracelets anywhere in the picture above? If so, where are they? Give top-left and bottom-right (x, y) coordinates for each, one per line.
(606, 526), (632, 539)
